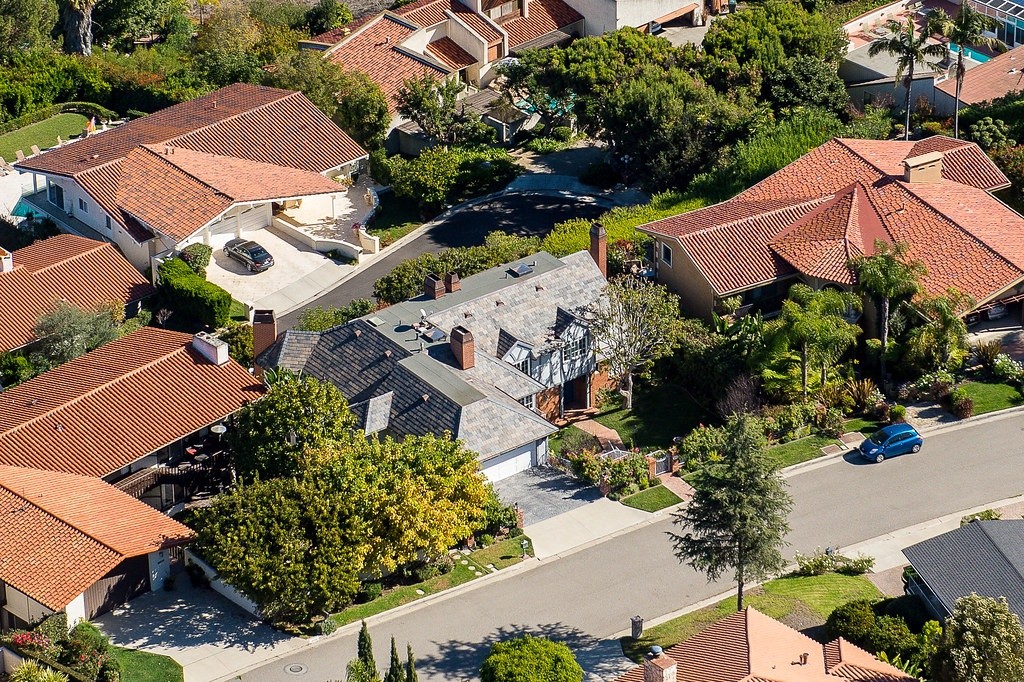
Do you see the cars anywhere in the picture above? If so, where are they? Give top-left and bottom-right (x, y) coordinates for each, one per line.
(222, 238), (275, 272)
(858, 423), (923, 463)
